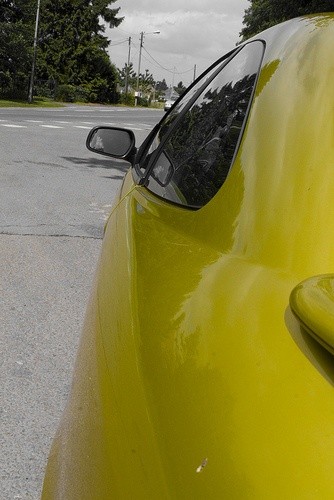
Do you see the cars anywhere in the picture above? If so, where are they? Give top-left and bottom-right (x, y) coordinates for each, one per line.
(41, 12), (333, 500)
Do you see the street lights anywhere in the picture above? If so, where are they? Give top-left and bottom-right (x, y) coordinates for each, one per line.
(132, 31), (161, 107)
